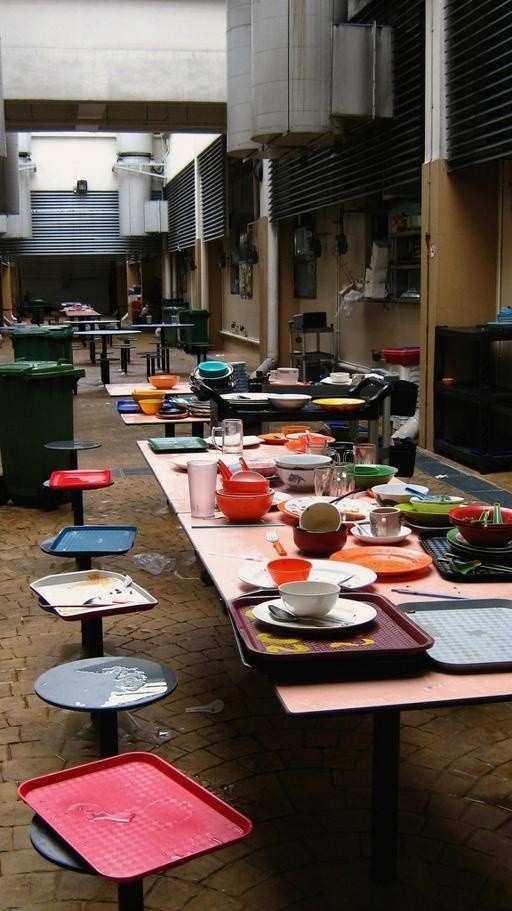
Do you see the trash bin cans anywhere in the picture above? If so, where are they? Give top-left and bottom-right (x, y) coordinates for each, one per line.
(0, 358), (86, 512)
(31, 299), (47, 324)
(8, 324), (79, 389)
(161, 307), (211, 354)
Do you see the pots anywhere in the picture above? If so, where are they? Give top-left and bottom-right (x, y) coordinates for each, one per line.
(402, 216), (420, 231)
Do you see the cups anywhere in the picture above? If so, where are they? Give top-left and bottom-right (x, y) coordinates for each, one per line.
(187, 460), (216, 520)
(370, 508), (402, 536)
(298, 503), (341, 533)
(209, 419), (244, 456)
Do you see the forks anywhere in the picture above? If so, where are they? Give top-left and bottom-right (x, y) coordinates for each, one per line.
(265, 526), (289, 557)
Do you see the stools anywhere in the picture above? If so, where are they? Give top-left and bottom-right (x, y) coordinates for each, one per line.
(42, 481), (116, 527)
(45, 440), (101, 473)
(35, 309), (215, 383)
(29, 814), (164, 910)
(34, 596), (122, 663)
(42, 535), (134, 576)
(35, 657), (179, 762)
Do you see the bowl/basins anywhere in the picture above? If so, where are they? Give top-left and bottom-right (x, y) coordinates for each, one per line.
(257, 419), (399, 500)
(448, 505), (511, 546)
(372, 484), (466, 533)
(216, 469), (275, 524)
(278, 583), (340, 619)
(292, 524), (348, 557)
(265, 559), (316, 584)
(132, 359), (365, 418)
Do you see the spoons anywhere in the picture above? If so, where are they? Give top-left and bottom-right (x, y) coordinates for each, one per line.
(268, 604), (347, 627)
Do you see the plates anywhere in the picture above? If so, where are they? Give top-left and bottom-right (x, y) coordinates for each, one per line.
(276, 495), (374, 527)
(350, 525), (412, 545)
(202, 434), (263, 448)
(148, 437), (211, 454)
(236, 559), (379, 592)
(447, 528), (511, 558)
(329, 546), (435, 577)
(251, 599), (378, 632)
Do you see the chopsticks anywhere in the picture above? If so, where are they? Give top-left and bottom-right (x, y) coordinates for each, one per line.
(437, 553), (511, 573)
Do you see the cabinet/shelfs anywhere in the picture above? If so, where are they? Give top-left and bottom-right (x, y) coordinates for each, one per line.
(428, 322), (511, 472)
(386, 197), (422, 304)
(288, 322), (336, 383)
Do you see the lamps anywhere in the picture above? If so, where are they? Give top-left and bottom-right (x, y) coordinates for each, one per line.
(75, 179), (88, 193)
